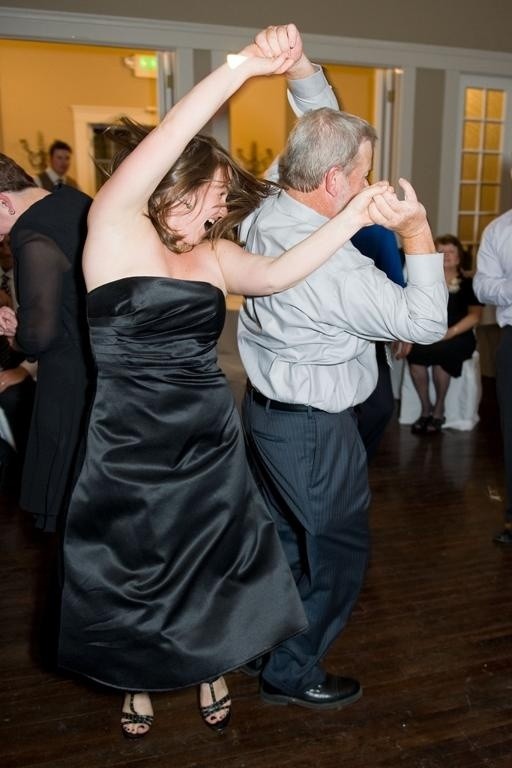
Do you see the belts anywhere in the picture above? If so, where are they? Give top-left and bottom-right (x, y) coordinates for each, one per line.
(246, 377), (318, 413)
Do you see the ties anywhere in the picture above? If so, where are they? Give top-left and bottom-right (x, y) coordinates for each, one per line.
(55, 178), (63, 187)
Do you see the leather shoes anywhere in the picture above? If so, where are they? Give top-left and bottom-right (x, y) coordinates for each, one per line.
(260, 670), (365, 711)
(492, 528), (511, 544)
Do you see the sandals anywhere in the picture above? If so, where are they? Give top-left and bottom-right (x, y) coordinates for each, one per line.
(198, 676), (232, 730)
(412, 415), (447, 436)
(119, 690), (154, 741)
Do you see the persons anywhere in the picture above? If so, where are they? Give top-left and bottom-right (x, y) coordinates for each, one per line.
(344, 210), (512, 545)
(0, 140), (100, 740)
(51, 25), (449, 739)
